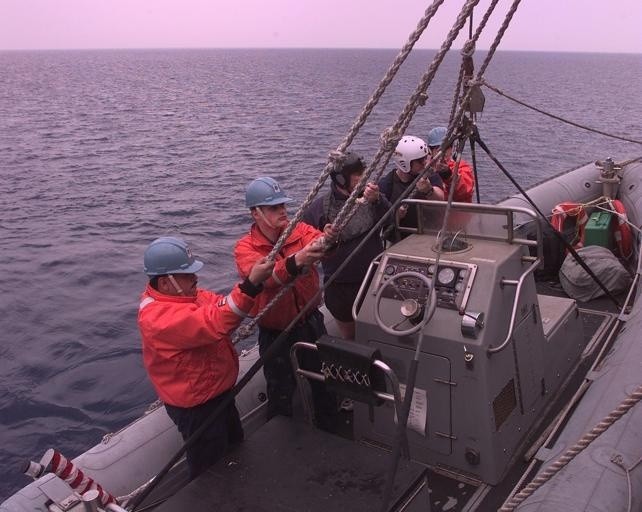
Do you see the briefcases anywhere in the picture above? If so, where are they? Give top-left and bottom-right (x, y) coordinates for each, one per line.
(584, 211), (614, 253)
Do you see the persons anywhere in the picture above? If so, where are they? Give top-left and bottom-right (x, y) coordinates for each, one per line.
(235, 176), (336, 422)
(303, 152), (393, 323)
(427, 127), (474, 203)
(378, 133), (444, 243)
(138, 236), (275, 478)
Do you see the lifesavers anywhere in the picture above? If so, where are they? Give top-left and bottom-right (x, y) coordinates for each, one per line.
(606, 198), (632, 259)
(551, 201), (589, 255)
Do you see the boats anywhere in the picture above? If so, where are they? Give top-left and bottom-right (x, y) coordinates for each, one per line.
(0, 157), (642, 512)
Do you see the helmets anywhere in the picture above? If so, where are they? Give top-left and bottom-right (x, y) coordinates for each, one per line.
(329, 150), (367, 190)
(427, 127), (449, 147)
(392, 134), (429, 174)
(245, 176), (293, 209)
(142, 236), (205, 276)
